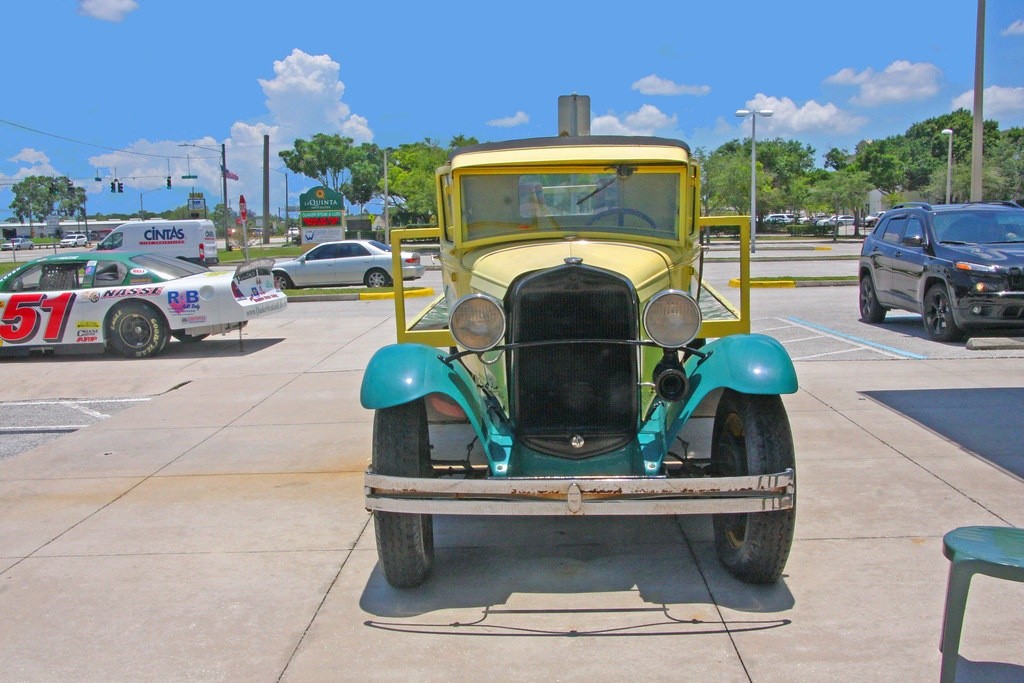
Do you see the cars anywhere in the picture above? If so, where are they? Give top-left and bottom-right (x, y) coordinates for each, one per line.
(288, 228), (301, 238)
(1, 253), (292, 362)
(797, 215), (812, 226)
(60, 234), (88, 248)
(0, 238), (36, 250)
(268, 238), (423, 287)
(816, 215), (839, 227)
(359, 135), (799, 589)
(860, 211), (886, 228)
(831, 215), (855, 227)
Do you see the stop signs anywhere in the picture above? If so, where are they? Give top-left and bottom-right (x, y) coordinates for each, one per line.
(238, 195), (248, 221)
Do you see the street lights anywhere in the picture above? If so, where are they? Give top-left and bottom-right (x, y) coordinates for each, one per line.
(941, 129), (954, 205)
(734, 109), (775, 252)
(260, 166), (289, 244)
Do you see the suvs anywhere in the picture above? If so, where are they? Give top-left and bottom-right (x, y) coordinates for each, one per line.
(858, 202), (1024, 345)
(763, 213), (797, 224)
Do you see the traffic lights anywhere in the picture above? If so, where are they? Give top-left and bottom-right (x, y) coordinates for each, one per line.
(111, 182), (115, 193)
(167, 177), (172, 190)
(118, 182), (124, 193)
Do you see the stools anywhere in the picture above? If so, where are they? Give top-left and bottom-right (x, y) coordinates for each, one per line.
(938, 524), (1024, 683)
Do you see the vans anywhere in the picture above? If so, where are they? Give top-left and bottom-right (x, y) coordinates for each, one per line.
(85, 220), (222, 268)
